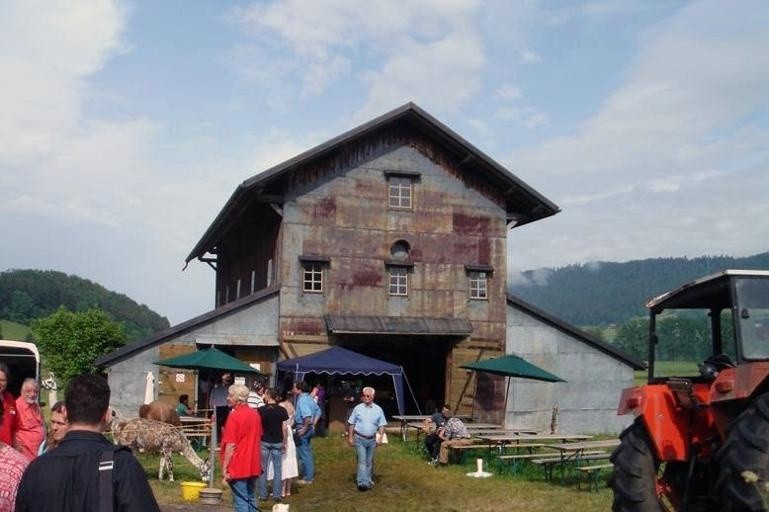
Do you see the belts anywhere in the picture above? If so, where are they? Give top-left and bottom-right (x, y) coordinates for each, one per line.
(354, 429), (376, 439)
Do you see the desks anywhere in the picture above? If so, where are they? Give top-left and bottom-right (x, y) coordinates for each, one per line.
(180, 416), (211, 446)
(392, 416), (620, 491)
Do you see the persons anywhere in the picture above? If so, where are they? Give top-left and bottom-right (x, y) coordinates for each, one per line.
(0, 361), (160, 512)
(175, 370), (327, 512)
(423, 404), (451, 464)
(347, 386), (388, 491)
(435, 412), (473, 467)
(341, 380), (361, 436)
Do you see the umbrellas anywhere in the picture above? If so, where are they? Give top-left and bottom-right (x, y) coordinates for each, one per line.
(152, 347), (259, 373)
(456, 351), (568, 427)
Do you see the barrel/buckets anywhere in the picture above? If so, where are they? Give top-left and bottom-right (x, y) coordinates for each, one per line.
(198, 488), (223, 505)
(179, 481), (208, 501)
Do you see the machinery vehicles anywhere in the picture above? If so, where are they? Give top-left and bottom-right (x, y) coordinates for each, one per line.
(604, 266), (769, 512)
